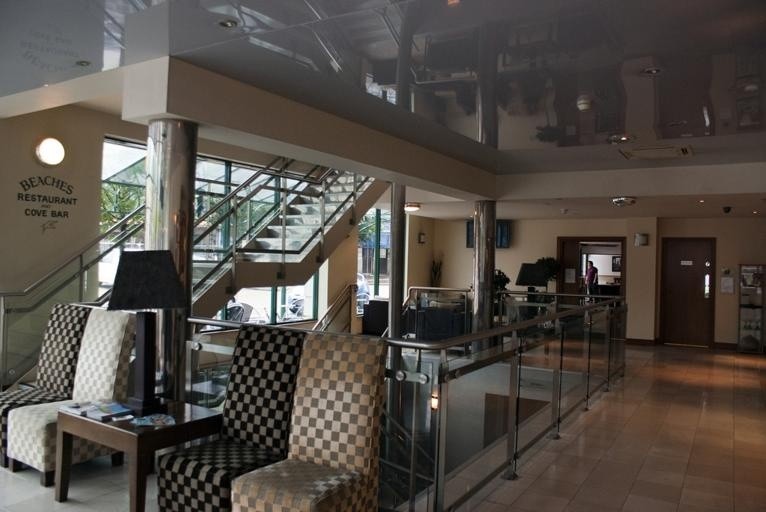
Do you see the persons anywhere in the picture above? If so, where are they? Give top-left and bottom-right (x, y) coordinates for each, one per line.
(586, 260), (600, 304)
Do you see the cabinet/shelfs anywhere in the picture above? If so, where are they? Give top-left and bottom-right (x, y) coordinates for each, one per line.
(506, 301), (556, 321)
(736, 262), (766, 355)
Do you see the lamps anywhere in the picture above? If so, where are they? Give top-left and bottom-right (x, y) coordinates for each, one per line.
(635, 232), (648, 247)
(35, 137), (66, 168)
(608, 197), (636, 208)
(419, 232), (425, 244)
(517, 262), (547, 303)
(404, 202), (424, 211)
(107, 249), (190, 416)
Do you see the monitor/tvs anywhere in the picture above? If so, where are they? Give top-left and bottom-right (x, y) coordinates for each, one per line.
(612, 256), (622, 272)
(466, 220), (512, 248)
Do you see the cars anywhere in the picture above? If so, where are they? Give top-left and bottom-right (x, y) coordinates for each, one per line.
(288, 271), (370, 314)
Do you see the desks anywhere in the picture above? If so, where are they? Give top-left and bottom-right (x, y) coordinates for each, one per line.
(56, 397), (229, 512)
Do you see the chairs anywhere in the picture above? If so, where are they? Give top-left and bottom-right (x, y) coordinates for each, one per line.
(8, 307), (135, 489)
(155, 322), (304, 512)
(231, 331), (391, 512)
(1, 302), (91, 469)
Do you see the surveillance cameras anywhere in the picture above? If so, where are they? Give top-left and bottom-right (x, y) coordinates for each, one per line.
(723, 207), (731, 213)
